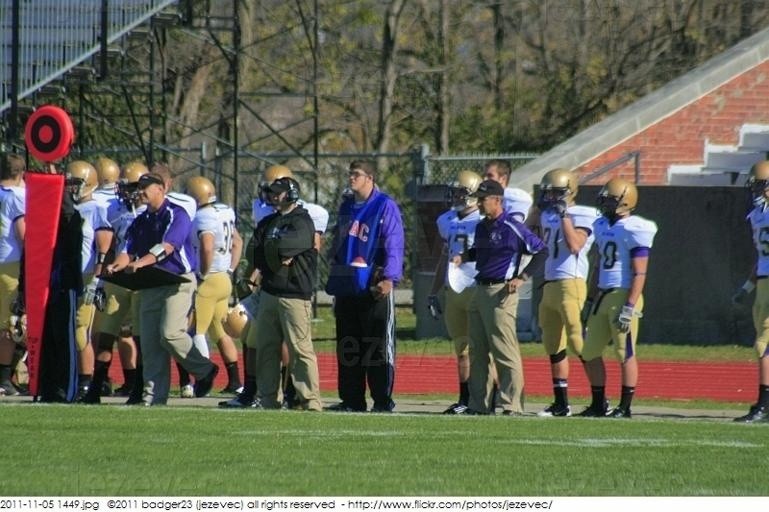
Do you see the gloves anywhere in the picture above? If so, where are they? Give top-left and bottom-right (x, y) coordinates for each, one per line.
(579, 299), (594, 323)
(552, 200), (566, 218)
(195, 272), (206, 287)
(426, 294), (443, 320)
(730, 288), (750, 310)
(613, 304), (634, 334)
(227, 268), (235, 276)
(82, 274), (108, 311)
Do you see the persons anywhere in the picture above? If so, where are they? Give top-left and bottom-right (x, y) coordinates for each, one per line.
(325, 158), (406, 412)
(733, 161), (768, 425)
(0, 153), (26, 397)
(93, 157), (122, 397)
(246, 178), (321, 412)
(79, 163), (150, 405)
(484, 161), (531, 226)
(218, 166), (329, 410)
(452, 181), (550, 417)
(59, 160), (113, 403)
(107, 174), (219, 406)
(524, 171), (602, 417)
(580, 176), (660, 420)
(429, 170), (500, 413)
(180, 177), (243, 398)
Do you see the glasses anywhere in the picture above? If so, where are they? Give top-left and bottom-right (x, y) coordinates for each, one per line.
(349, 171), (369, 177)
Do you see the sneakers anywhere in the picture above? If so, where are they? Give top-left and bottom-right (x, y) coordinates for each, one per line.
(571, 400), (611, 416)
(1, 381), (29, 397)
(216, 390), (257, 409)
(535, 404), (573, 417)
(124, 394), (148, 405)
(441, 403), (468, 414)
(603, 407), (632, 419)
(194, 363), (220, 399)
(180, 380), (195, 398)
(731, 403), (769, 423)
(115, 380), (136, 396)
(223, 383), (244, 394)
(370, 400), (395, 413)
(321, 401), (368, 412)
(76, 393), (101, 405)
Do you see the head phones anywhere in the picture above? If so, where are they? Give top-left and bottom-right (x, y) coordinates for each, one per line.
(265, 177), (299, 208)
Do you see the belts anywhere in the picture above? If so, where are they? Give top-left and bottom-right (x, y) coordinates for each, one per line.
(473, 278), (509, 286)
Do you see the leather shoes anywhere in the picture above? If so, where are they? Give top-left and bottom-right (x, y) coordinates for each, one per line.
(458, 407), (491, 416)
(502, 409), (522, 416)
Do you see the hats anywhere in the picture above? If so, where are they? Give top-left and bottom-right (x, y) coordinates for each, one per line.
(468, 180), (504, 198)
(132, 172), (166, 187)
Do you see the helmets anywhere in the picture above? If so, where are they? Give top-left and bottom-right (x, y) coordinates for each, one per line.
(185, 176), (217, 207)
(595, 176), (638, 216)
(444, 170), (483, 212)
(91, 158), (120, 185)
(256, 165), (295, 209)
(265, 177), (301, 207)
(745, 160), (768, 209)
(113, 163), (150, 213)
(63, 161), (101, 203)
(220, 310), (252, 340)
(537, 169), (577, 209)
